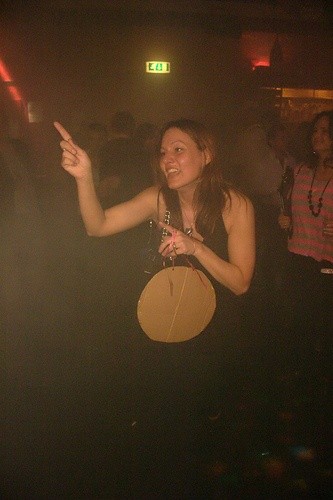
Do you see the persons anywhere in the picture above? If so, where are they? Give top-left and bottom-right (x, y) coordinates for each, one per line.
(236, 110), (333, 460)
(0, 112), (165, 258)
(54, 119), (255, 500)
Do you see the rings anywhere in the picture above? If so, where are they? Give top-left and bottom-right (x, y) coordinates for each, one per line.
(173, 244), (176, 250)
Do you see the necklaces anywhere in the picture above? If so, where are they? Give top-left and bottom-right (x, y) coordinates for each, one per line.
(183, 207), (196, 237)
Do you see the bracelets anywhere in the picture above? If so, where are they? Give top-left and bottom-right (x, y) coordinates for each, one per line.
(192, 239), (196, 255)
